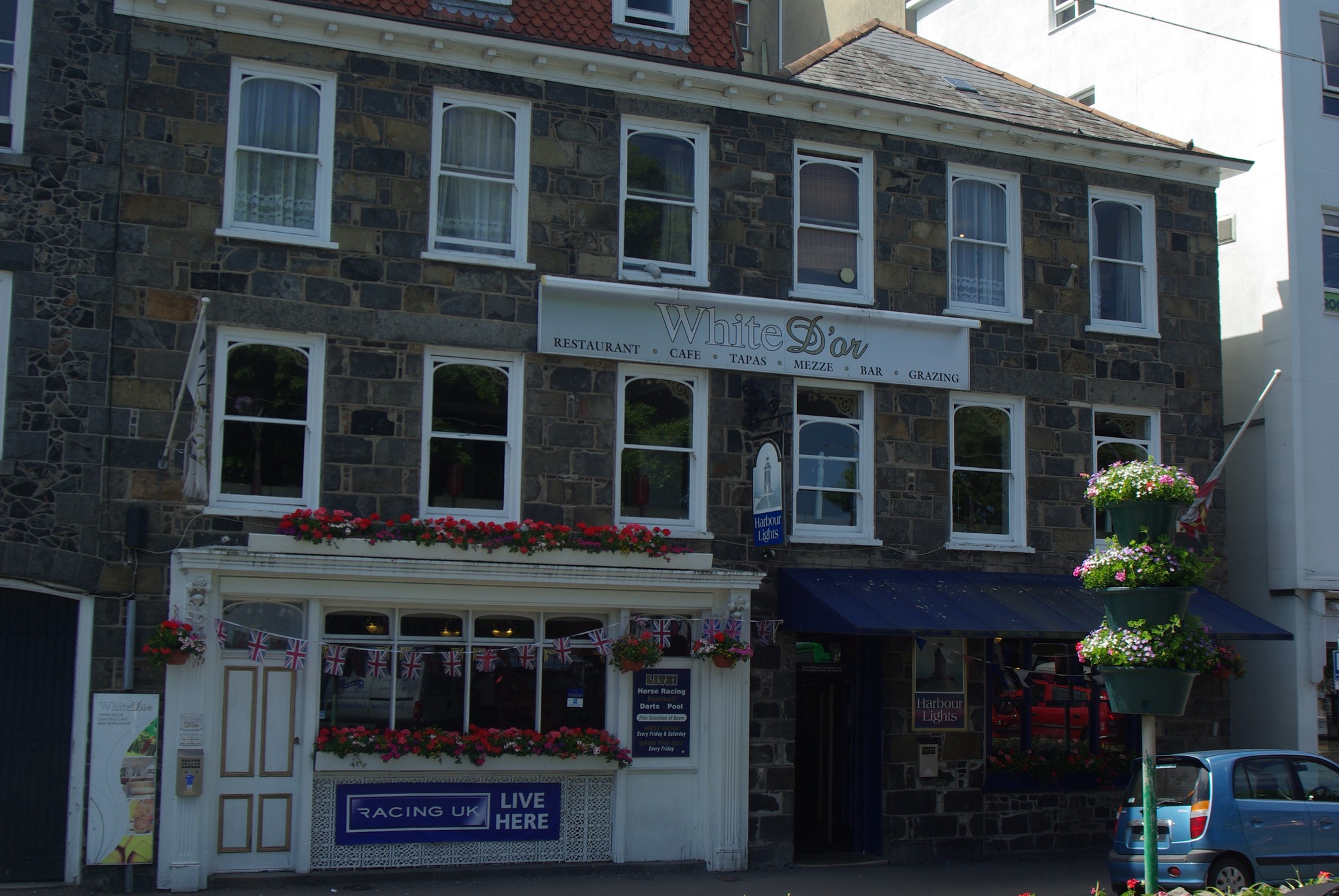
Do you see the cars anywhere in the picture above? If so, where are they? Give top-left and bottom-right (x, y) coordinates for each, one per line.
(1107, 749), (1339, 896)
(991, 680), (1128, 748)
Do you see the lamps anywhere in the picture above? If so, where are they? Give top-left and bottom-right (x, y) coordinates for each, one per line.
(365, 625), (387, 634)
(491, 629), (513, 638)
(439, 631), (460, 637)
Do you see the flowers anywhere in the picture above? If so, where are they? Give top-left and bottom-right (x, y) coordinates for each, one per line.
(309, 723), (632, 769)
(277, 507), (694, 563)
(1071, 457), (1248, 679)
(142, 619), (207, 669)
(689, 631), (754, 670)
(988, 736), (1140, 791)
(608, 630), (663, 674)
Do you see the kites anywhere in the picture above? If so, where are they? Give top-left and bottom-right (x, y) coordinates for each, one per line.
(401, 652), (422, 680)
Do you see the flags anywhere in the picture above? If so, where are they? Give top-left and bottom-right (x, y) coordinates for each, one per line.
(214, 618), (227, 650)
(442, 652), (462, 676)
(588, 628), (612, 656)
(724, 619), (743, 641)
(702, 619), (720, 640)
(517, 644), (535, 670)
(631, 615), (648, 628)
(476, 649), (497, 672)
(756, 621), (775, 646)
(324, 644), (348, 675)
(552, 637), (573, 664)
(653, 620), (671, 648)
(367, 650), (388, 679)
(248, 629), (270, 663)
(284, 638), (308, 671)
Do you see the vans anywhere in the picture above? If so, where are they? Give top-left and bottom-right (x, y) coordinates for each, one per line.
(322, 646), (456, 731)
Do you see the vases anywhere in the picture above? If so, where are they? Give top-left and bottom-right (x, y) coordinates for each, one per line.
(620, 657), (644, 672)
(1211, 666), (1232, 679)
(1106, 500), (1183, 547)
(711, 654), (737, 668)
(163, 646), (188, 665)
(314, 750), (619, 771)
(1095, 586), (1198, 636)
(1096, 665), (1200, 715)
